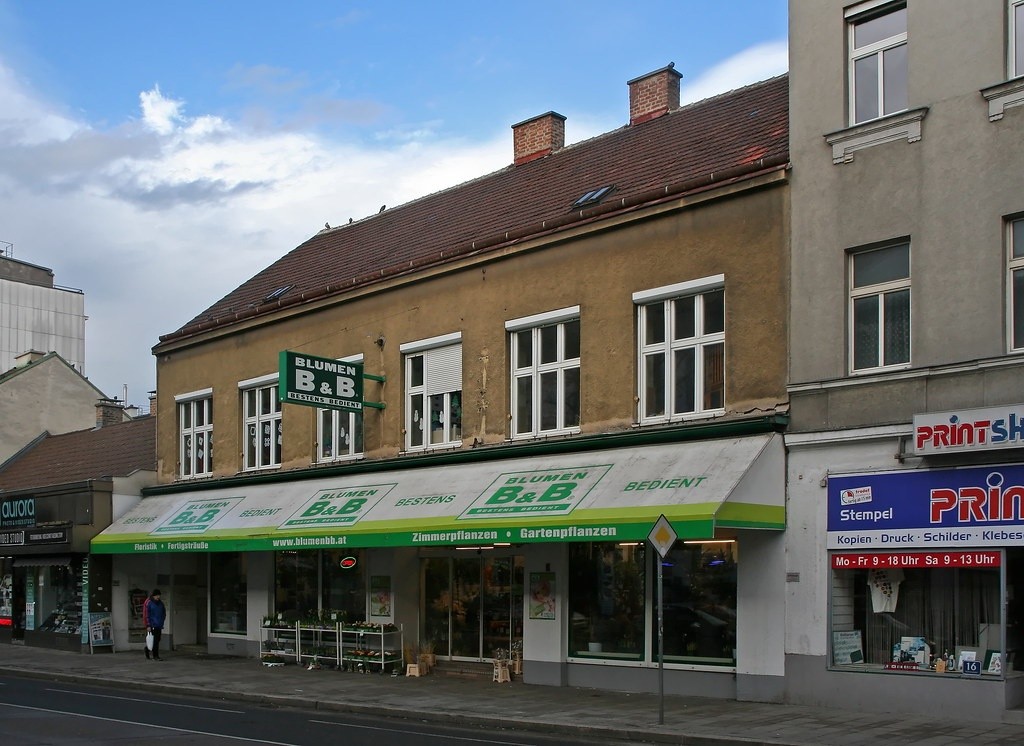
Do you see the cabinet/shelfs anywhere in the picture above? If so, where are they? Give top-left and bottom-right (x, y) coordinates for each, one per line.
(298, 620), (358, 671)
(339, 622), (405, 676)
(258, 619), (320, 664)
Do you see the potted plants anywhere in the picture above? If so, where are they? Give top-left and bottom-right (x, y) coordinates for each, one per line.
(403, 636), (438, 677)
(492, 647), (511, 683)
(511, 640), (523, 675)
(260, 608), (402, 675)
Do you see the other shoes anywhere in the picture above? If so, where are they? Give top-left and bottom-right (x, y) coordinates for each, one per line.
(144, 648), (150, 660)
(154, 657), (163, 661)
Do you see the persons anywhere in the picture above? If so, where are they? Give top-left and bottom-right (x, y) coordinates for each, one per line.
(143, 589), (166, 661)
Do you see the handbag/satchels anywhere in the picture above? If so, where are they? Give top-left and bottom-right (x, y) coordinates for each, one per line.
(146, 630), (154, 651)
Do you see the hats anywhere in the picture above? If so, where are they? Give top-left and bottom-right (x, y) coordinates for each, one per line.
(151, 589), (161, 596)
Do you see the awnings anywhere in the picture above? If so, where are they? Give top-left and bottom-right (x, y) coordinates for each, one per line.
(89, 431), (786, 554)
